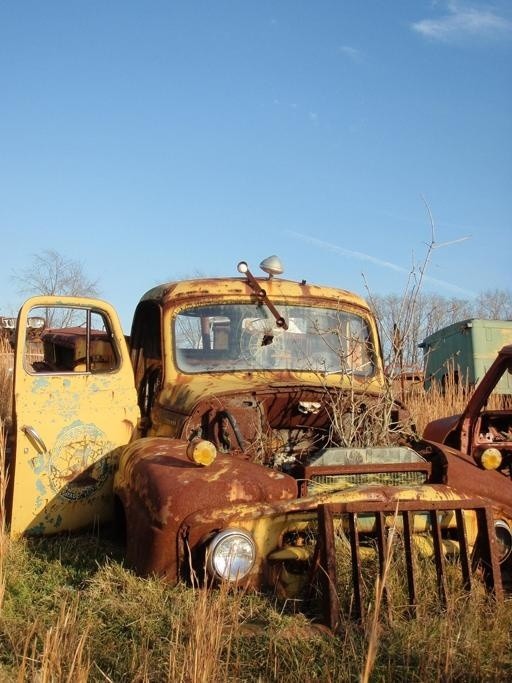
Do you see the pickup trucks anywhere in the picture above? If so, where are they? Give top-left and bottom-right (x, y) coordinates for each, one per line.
(6, 278), (512, 624)
